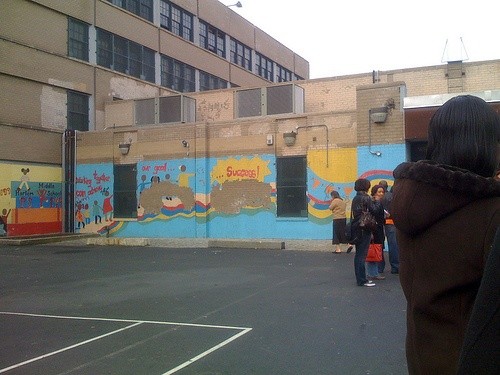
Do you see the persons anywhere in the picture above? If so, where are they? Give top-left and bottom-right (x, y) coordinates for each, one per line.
(350, 178), (402, 287)
(392, 95), (500, 375)
(329, 191), (353, 254)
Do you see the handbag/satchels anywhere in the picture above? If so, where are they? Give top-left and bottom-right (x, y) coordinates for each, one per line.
(365, 238), (382, 262)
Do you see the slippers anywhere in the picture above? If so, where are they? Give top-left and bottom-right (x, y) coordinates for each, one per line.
(347, 246), (353, 253)
(332, 250), (341, 253)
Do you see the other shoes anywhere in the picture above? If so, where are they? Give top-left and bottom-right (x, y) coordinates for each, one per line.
(378, 270), (383, 273)
(391, 271), (398, 274)
(360, 280), (375, 287)
(367, 276), (385, 280)
